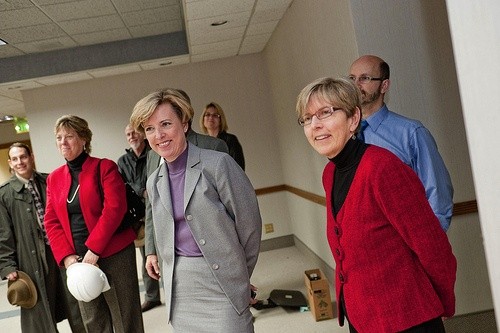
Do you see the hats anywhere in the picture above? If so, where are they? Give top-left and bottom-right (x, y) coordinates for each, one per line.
(7, 271), (37, 308)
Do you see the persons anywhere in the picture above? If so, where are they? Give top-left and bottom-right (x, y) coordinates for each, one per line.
(295, 75), (458, 333)
(0, 89), (229, 333)
(200, 101), (246, 173)
(129, 89), (262, 333)
(43, 114), (146, 333)
(345, 55), (454, 234)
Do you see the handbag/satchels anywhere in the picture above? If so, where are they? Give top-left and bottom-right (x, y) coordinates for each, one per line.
(97, 158), (147, 230)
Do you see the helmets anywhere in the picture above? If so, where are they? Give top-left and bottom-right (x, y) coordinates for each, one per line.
(66, 263), (111, 302)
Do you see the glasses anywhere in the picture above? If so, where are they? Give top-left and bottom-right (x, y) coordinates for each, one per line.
(298, 105), (344, 127)
(204, 112), (220, 118)
(346, 74), (385, 85)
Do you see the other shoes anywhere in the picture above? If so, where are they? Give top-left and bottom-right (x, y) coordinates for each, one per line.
(141, 300), (161, 313)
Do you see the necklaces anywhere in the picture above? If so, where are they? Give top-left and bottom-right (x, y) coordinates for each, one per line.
(66, 184), (80, 203)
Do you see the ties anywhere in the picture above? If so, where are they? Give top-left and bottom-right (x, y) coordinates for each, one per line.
(27, 180), (50, 245)
